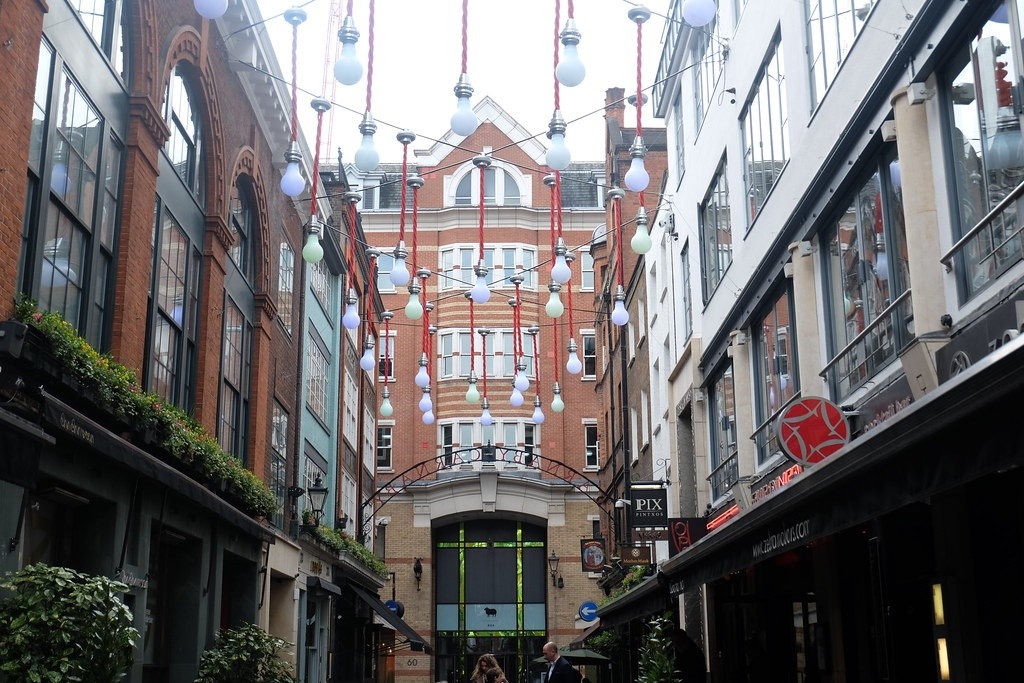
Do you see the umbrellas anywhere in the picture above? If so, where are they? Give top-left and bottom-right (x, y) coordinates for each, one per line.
(532, 649), (611, 665)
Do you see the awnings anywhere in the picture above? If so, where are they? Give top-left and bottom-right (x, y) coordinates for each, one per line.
(569, 620), (600, 644)
(346, 582), (433, 656)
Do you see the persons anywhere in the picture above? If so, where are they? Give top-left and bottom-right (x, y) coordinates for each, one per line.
(542, 641), (572, 683)
(486, 668), (508, 683)
(470, 654), (505, 683)
(586, 547), (596, 566)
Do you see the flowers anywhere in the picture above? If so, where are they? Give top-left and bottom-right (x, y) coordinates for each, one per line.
(303, 511), (389, 579)
(12, 297), (278, 519)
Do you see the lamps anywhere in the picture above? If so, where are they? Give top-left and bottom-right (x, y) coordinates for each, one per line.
(413, 557), (421, 592)
(547, 549), (559, 587)
(299, 471), (329, 529)
